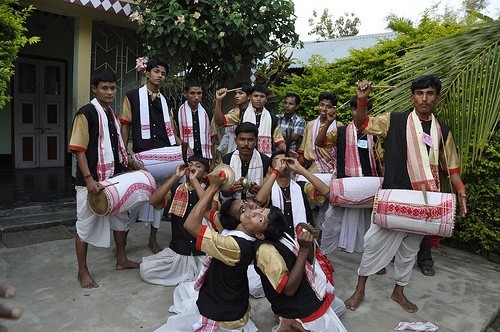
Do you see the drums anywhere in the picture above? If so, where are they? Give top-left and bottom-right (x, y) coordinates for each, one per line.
(132, 145), (194, 181)
(87, 169), (157, 216)
(330, 176), (384, 209)
(373, 187), (456, 237)
(295, 173), (333, 186)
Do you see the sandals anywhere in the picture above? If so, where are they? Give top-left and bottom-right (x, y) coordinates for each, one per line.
(420, 265), (434, 275)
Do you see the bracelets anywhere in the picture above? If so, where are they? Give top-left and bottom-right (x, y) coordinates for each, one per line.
(83, 174), (91, 181)
(357, 98), (369, 105)
(272, 169), (281, 179)
(456, 184), (465, 191)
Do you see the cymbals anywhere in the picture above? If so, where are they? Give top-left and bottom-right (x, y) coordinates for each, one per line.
(214, 164), (236, 191)
(242, 173), (252, 194)
(295, 222), (316, 239)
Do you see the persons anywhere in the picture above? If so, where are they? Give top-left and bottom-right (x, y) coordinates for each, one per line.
(220, 122), (273, 206)
(173, 81), (387, 275)
(0, 282), (22, 332)
(344, 75), (467, 314)
(67, 70), (149, 289)
(112, 60), (184, 255)
(153, 170), (287, 332)
(219, 196), (349, 332)
(139, 155), (221, 286)
(247, 150), (331, 298)
(418, 235), (435, 276)
